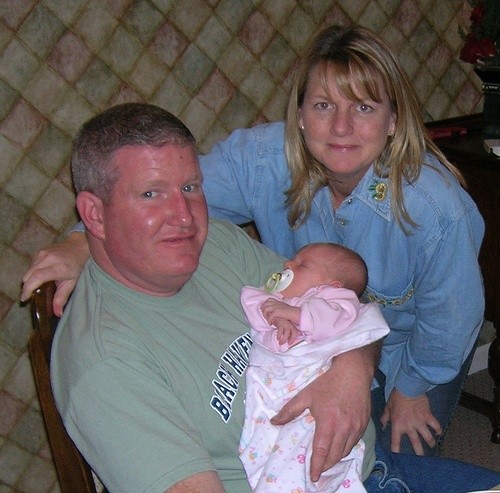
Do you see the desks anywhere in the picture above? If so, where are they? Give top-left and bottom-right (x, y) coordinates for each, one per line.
(423, 115), (500, 442)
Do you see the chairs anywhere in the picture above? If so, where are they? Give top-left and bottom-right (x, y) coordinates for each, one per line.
(27, 281), (96, 489)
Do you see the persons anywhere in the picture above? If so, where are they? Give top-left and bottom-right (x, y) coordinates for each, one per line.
(21, 25), (485, 458)
(239, 242), (368, 493)
(50, 103), (500, 493)
